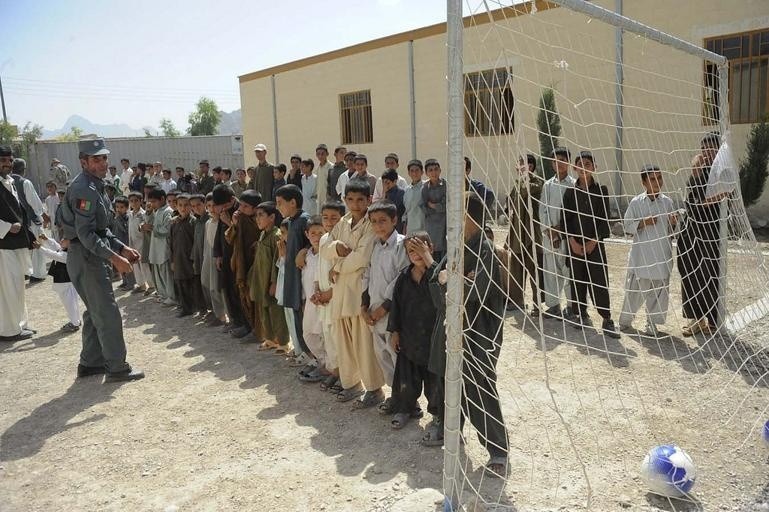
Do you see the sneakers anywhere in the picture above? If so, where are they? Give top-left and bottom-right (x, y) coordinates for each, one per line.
(574, 317), (705, 338)
(61, 323), (79, 331)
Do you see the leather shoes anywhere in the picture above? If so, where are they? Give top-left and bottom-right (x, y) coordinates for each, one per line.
(24, 327), (38, 334)
(105, 369), (145, 383)
(2, 330), (34, 341)
(78, 364), (106, 377)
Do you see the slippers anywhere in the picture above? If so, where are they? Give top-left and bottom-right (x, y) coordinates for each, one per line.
(117, 281), (226, 327)
(229, 326), (290, 356)
(507, 306), (575, 321)
(285, 350), (385, 409)
(377, 399), (508, 477)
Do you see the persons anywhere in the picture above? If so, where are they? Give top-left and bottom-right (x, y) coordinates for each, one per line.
(62, 138), (144, 383)
(504, 155), (545, 317)
(677, 132), (726, 336)
(107, 142), (492, 431)
(0, 148), (82, 341)
(619, 165), (681, 334)
(560, 152), (622, 338)
(539, 147), (576, 321)
(422, 191), (511, 477)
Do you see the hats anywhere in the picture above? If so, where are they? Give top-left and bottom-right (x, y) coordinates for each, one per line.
(12, 158), (27, 170)
(78, 137), (111, 156)
(0, 144), (13, 156)
(255, 143), (267, 151)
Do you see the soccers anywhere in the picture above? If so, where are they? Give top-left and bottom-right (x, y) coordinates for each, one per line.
(642, 444), (695, 497)
